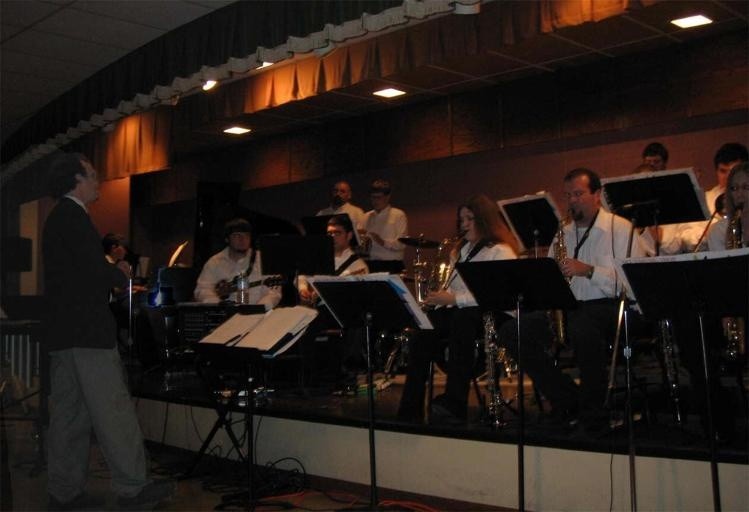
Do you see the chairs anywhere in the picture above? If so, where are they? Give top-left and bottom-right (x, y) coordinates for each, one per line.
(423, 339), (545, 427)
(555, 322), (660, 432)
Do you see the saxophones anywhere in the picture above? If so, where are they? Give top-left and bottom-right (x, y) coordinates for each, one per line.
(721, 202), (745, 360)
(550, 206), (572, 342)
(414, 231), (468, 307)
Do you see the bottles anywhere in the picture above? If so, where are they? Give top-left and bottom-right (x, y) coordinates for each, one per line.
(486, 392), (507, 428)
(236, 266), (249, 304)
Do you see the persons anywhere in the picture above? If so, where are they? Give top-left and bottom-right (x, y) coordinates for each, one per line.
(102, 232), (158, 341)
(503, 138), (749, 447)
(314, 179), (363, 223)
(353, 180), (408, 274)
(40, 150), (173, 512)
(193, 218), (281, 399)
(117, 239), (144, 283)
(294, 218), (369, 397)
(395, 195), (515, 425)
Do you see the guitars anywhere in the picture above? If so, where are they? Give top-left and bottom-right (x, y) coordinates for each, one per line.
(214, 276), (281, 298)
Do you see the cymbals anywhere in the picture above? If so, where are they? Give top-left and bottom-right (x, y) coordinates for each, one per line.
(396, 234), (439, 248)
